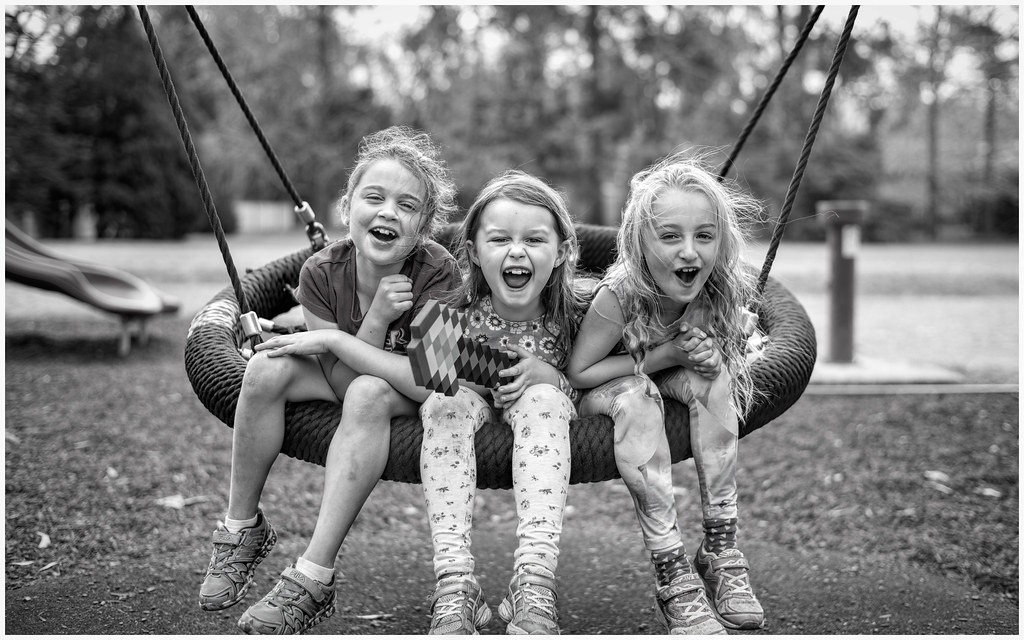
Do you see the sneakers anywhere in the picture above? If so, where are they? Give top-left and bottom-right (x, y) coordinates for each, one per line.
(498, 573), (559, 633)
(238, 565), (338, 635)
(198, 506), (278, 611)
(427, 570), (490, 635)
(696, 538), (764, 629)
(655, 568), (728, 635)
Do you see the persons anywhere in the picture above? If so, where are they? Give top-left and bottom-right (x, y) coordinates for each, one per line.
(198, 126), (471, 635)
(568, 162), (764, 635)
(419, 174), (593, 635)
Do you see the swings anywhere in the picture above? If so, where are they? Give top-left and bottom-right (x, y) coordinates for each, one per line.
(137, 6), (864, 489)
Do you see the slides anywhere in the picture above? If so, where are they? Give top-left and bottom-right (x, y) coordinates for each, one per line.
(5, 219), (185, 320)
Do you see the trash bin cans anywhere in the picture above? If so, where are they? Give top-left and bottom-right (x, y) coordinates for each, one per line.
(72, 202), (97, 239)
